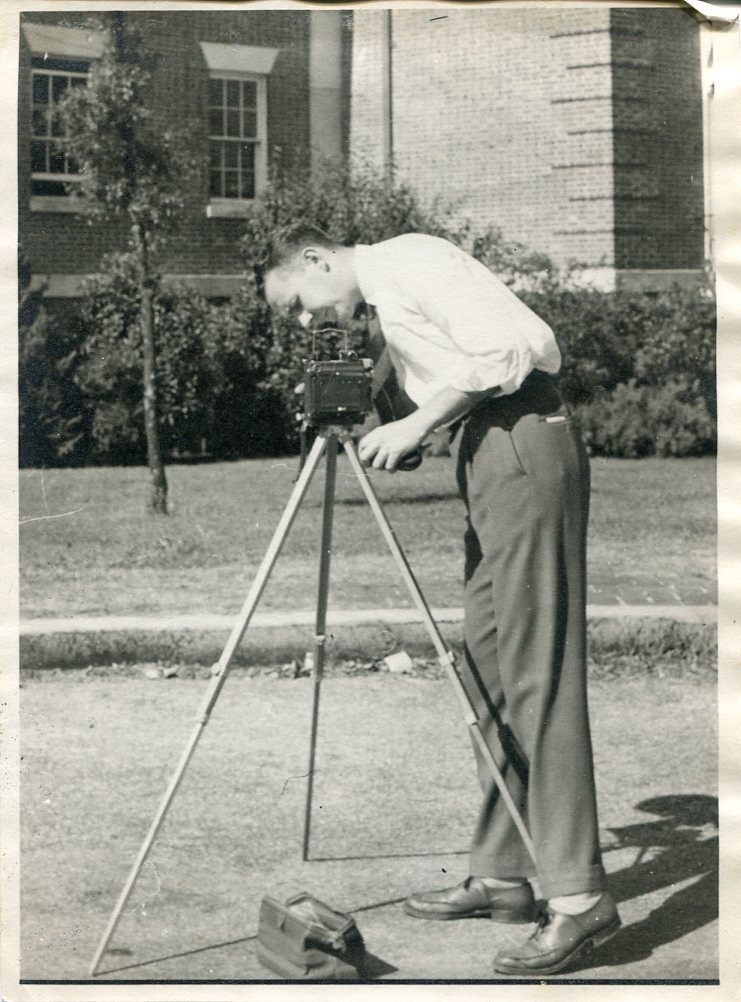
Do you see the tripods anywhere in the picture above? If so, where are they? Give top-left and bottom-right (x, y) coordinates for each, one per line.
(87, 426), (538, 977)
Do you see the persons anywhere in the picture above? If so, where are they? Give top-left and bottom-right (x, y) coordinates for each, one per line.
(253, 226), (620, 977)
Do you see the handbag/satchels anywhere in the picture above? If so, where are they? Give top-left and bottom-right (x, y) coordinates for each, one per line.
(256, 892), (365, 979)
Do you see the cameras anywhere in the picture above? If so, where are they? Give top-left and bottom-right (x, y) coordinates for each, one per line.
(304, 322), (374, 425)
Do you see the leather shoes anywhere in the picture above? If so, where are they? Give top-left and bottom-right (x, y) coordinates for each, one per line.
(403, 876), (535, 924)
(493, 890), (621, 975)
